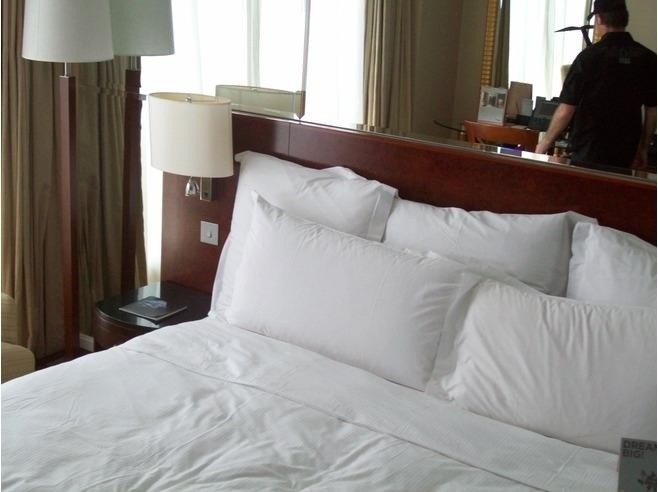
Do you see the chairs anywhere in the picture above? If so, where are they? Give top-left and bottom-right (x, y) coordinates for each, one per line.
(462, 118), (540, 155)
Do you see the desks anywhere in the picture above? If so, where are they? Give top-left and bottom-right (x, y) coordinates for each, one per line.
(433, 115), (572, 153)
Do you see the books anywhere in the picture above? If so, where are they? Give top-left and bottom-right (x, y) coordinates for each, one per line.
(117, 294), (189, 323)
(616, 434), (657, 492)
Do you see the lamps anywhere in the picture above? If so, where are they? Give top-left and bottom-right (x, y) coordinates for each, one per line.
(107, 1), (177, 307)
(215, 82), (306, 120)
(16, 1), (116, 357)
(148, 91), (237, 203)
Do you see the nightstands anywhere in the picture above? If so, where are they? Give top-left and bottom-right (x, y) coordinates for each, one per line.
(90, 281), (209, 348)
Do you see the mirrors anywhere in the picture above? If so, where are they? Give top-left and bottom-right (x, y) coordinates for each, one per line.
(76, 0), (654, 301)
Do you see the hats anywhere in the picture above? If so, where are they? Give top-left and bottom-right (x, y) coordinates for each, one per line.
(586, 0), (628, 21)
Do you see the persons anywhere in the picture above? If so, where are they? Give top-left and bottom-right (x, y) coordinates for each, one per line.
(529, 0), (657, 172)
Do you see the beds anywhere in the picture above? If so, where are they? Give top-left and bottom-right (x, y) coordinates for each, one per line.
(1, 313), (655, 492)
